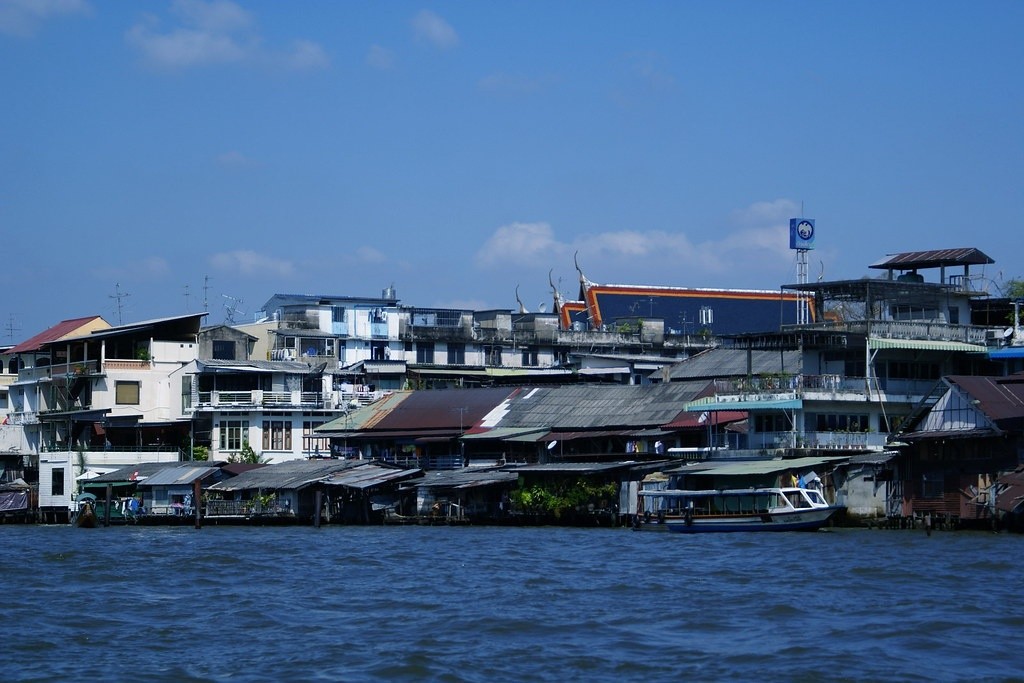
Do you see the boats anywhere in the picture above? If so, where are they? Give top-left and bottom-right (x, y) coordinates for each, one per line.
(631, 487), (851, 536)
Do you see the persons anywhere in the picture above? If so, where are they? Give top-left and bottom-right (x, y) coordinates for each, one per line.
(384, 344), (391, 359)
(925, 511), (931, 536)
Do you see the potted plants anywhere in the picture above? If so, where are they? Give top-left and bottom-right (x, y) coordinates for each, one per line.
(74, 364), (85, 375)
(797, 421), (876, 449)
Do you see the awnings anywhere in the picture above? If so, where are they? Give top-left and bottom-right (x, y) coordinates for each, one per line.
(36, 408), (112, 422)
(303, 427), (551, 460)
(397, 470), (518, 488)
(348, 359), (408, 374)
(686, 399), (803, 412)
(538, 428), (677, 444)
(83, 480), (135, 488)
(408, 366), (572, 381)
(989, 348), (1024, 359)
(868, 338), (987, 352)
(67, 372), (108, 389)
